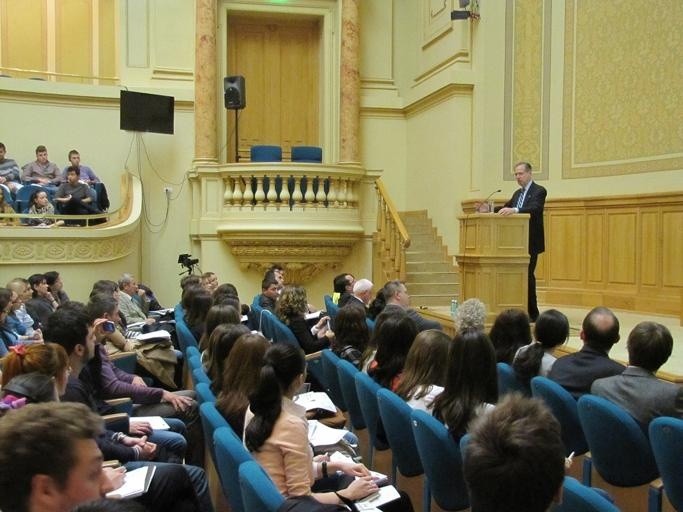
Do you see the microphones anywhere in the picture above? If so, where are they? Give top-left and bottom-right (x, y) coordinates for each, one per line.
(476, 188), (503, 212)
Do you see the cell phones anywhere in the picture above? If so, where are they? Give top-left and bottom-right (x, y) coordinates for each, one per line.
(101, 321), (115, 332)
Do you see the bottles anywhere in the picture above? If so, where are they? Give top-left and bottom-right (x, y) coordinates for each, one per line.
(487, 195), (495, 213)
(450, 298), (458, 314)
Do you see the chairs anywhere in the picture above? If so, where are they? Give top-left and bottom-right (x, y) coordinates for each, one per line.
(14, 183), (54, 213)
(287, 146), (329, 204)
(0, 184), (14, 209)
(53, 187), (97, 222)
(250, 146), (283, 202)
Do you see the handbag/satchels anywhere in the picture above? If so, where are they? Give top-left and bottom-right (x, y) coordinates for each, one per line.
(306, 406), (346, 429)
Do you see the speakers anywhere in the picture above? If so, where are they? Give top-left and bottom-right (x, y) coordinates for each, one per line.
(224, 75), (246, 109)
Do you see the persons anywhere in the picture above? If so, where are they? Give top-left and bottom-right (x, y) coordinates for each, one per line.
(52, 165), (108, 227)
(61, 149), (100, 188)
(0, 187), (22, 227)
(473, 162), (547, 324)
(0, 266), (682, 512)
(19, 189), (66, 229)
(0, 139), (21, 203)
(21, 144), (63, 189)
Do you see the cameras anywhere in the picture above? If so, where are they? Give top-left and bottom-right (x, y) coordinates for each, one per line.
(177, 252), (199, 269)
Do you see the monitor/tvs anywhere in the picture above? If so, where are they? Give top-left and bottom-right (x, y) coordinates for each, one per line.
(120, 89), (178, 135)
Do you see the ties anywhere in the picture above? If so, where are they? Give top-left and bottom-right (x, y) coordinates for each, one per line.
(518, 189), (524, 209)
